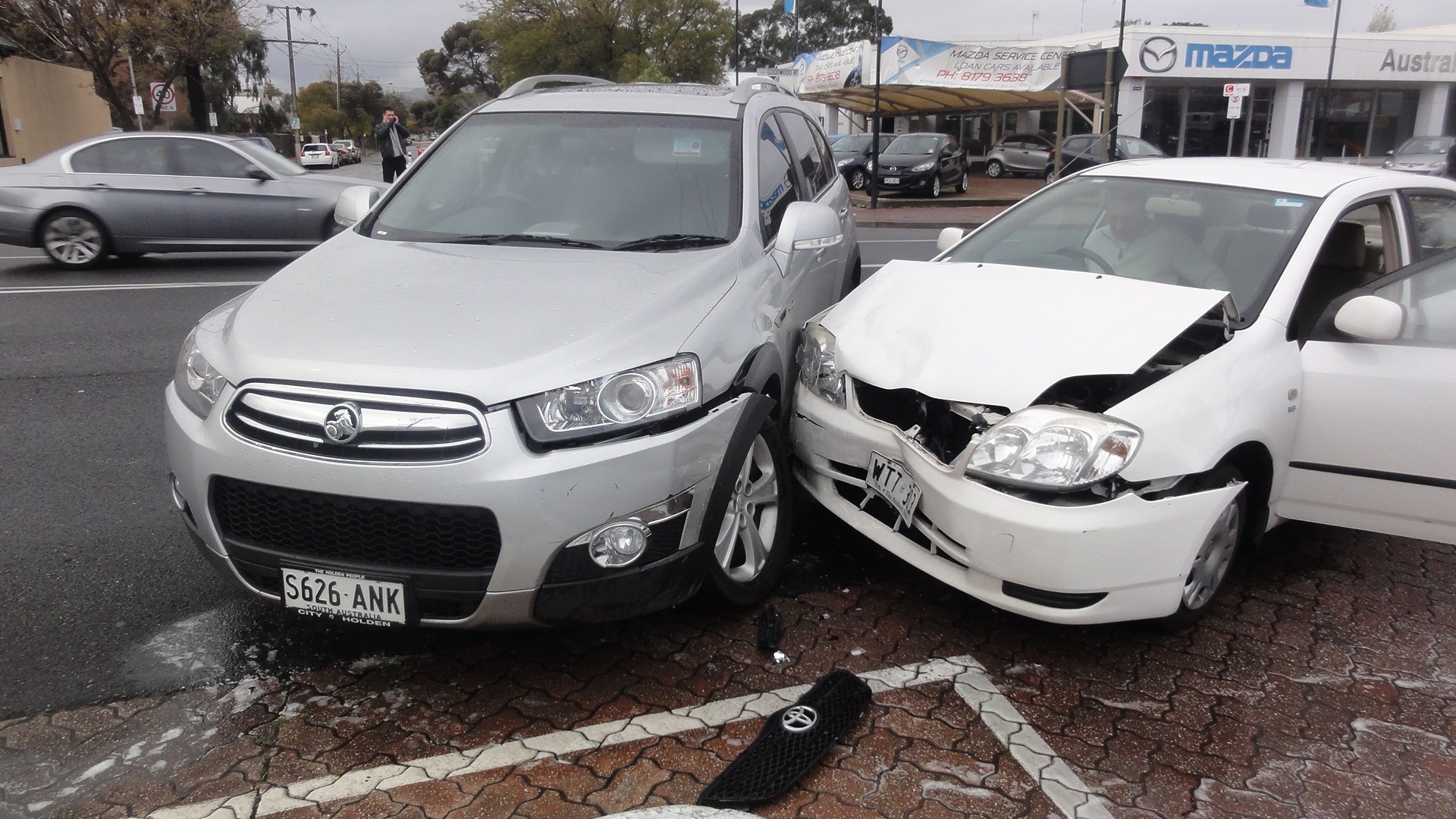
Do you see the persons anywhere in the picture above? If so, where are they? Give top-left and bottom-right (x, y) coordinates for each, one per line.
(375, 107), (410, 184)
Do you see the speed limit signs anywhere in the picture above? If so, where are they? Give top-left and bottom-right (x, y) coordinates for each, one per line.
(152, 84), (175, 104)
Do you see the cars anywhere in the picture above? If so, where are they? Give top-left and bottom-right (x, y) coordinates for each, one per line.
(0, 130), (398, 270)
(300, 142), (340, 169)
(430, 134), (439, 140)
(788, 156), (1456, 644)
(405, 133), (418, 145)
(1381, 136), (1456, 178)
(862, 131), (972, 198)
(829, 132), (900, 190)
(414, 141), (434, 156)
(826, 133), (849, 146)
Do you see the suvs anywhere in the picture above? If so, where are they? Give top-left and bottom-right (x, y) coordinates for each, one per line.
(983, 131), (1080, 179)
(161, 69), (861, 637)
(1043, 133), (1171, 184)
(328, 137), (363, 166)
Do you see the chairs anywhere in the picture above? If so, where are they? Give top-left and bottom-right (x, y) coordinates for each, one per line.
(1223, 201), (1296, 306)
(1146, 197), (1202, 219)
(1293, 222), (1377, 340)
